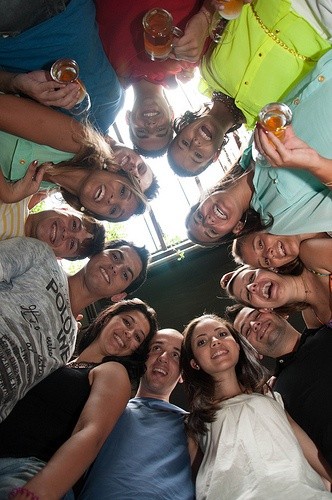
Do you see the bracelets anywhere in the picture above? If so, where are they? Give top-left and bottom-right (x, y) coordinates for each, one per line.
(322, 182), (332, 186)
(8, 487), (39, 500)
(201, 7), (211, 24)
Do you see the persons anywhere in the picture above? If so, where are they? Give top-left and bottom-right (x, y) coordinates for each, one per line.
(0, 0), (332, 500)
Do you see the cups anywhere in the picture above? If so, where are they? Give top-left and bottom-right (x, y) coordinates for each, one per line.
(143, 8), (184, 61)
(50, 58), (91, 115)
(252, 103), (292, 167)
(209, 0), (244, 44)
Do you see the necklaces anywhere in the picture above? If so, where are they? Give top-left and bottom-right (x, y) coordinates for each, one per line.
(300, 274), (308, 306)
(212, 91), (242, 128)
